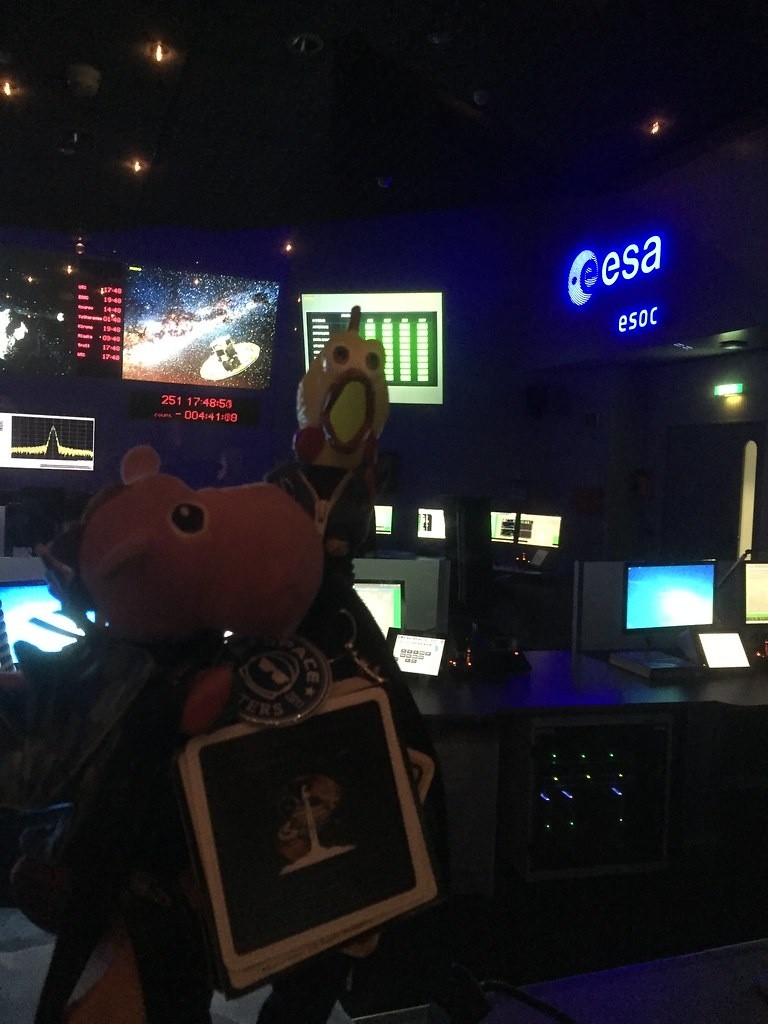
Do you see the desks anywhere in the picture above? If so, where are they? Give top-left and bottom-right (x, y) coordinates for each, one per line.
(396, 648), (768, 716)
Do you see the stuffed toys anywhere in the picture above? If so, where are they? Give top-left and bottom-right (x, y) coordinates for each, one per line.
(0, 446), (321, 1024)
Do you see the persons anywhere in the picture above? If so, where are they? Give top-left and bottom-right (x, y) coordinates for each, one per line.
(127, 465), (443, 1021)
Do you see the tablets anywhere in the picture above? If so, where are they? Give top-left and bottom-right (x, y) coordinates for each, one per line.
(697, 633), (751, 669)
(393, 634), (446, 676)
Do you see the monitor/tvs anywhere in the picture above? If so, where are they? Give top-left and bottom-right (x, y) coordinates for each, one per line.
(375, 506), (392, 535)
(490, 512), (562, 548)
(742, 561), (768, 626)
(0, 580), (97, 671)
(417, 508), (446, 539)
(353, 580), (405, 640)
(622, 560), (718, 629)
